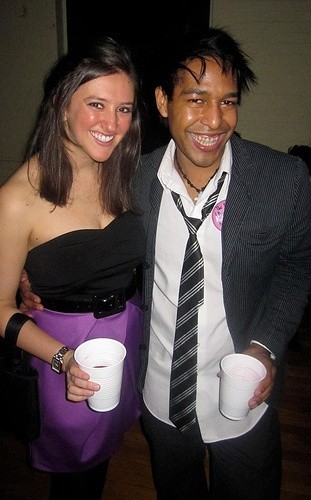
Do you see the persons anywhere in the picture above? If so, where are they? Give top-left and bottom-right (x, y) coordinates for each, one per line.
(0, 33), (158, 500)
(17, 27), (311, 500)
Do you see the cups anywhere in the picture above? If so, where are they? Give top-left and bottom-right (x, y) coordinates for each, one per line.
(218, 353), (267, 421)
(73, 337), (127, 412)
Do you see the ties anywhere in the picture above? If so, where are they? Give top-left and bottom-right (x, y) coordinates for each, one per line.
(169, 171), (227, 434)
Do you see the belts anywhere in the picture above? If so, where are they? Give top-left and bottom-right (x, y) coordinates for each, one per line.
(35, 275), (136, 319)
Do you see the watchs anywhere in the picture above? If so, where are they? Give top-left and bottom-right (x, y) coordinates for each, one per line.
(51, 346), (71, 375)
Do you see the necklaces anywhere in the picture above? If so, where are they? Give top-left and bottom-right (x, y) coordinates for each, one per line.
(174, 147), (220, 201)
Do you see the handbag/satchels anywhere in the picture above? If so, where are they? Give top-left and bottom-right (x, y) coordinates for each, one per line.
(0, 313), (40, 441)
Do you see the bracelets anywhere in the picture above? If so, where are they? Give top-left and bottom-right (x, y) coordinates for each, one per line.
(269, 351), (275, 364)
